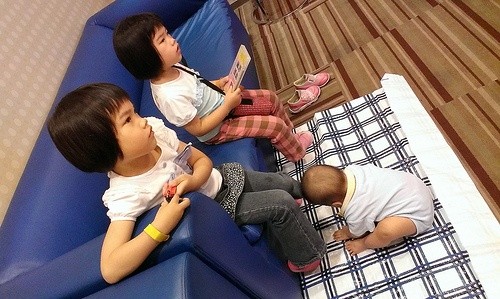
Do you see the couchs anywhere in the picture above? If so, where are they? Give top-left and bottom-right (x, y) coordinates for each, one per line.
(1, 0), (303, 299)
